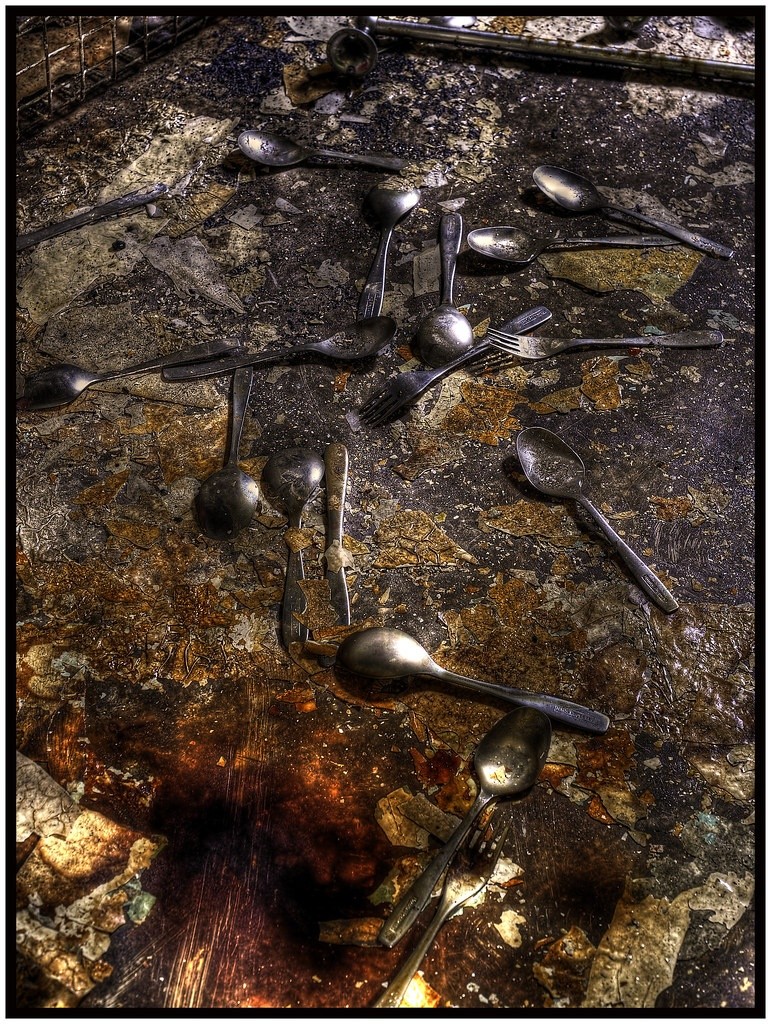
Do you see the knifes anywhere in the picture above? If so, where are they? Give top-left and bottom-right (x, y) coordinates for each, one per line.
(320, 440), (351, 668)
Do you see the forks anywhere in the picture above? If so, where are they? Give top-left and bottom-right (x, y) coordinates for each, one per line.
(373, 804), (512, 1006)
(357, 306), (553, 428)
(484, 326), (723, 358)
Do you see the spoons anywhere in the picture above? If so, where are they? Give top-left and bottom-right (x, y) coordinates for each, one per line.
(532, 164), (735, 258)
(192, 366), (259, 539)
(516, 424), (681, 613)
(261, 446), (326, 650)
(23, 335), (239, 409)
(416, 211), (473, 369)
(333, 630), (611, 736)
(356, 180), (421, 317)
(236, 130), (449, 174)
(376, 706), (550, 949)
(467, 225), (681, 261)
(161, 316), (397, 381)
